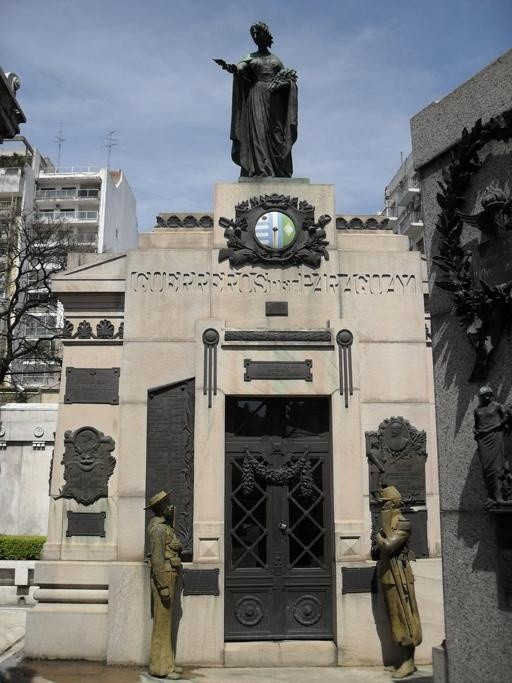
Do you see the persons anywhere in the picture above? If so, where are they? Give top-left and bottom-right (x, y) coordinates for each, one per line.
(213, 20), (298, 177)
(144, 490), (184, 680)
(387, 423), (408, 449)
(376, 485), (422, 678)
(473, 386), (509, 509)
